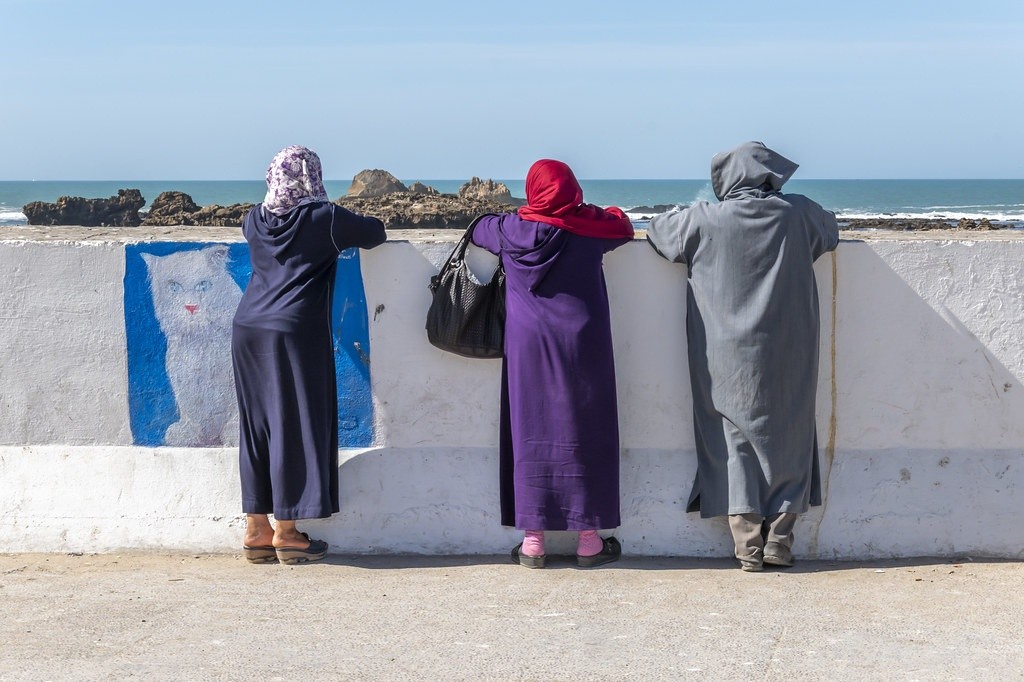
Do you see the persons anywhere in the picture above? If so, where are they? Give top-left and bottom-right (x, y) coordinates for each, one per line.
(231, 145), (387, 564)
(469, 160), (634, 567)
(646, 141), (838, 575)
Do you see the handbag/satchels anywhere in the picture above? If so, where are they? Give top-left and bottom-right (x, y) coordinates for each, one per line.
(426, 213), (506, 359)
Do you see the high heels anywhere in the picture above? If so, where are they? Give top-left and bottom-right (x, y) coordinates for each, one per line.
(276, 532), (328, 565)
(512, 542), (546, 568)
(243, 546), (277, 563)
(577, 536), (621, 566)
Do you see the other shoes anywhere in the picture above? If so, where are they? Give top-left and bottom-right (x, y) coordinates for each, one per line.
(763, 542), (793, 566)
(740, 559), (764, 571)
